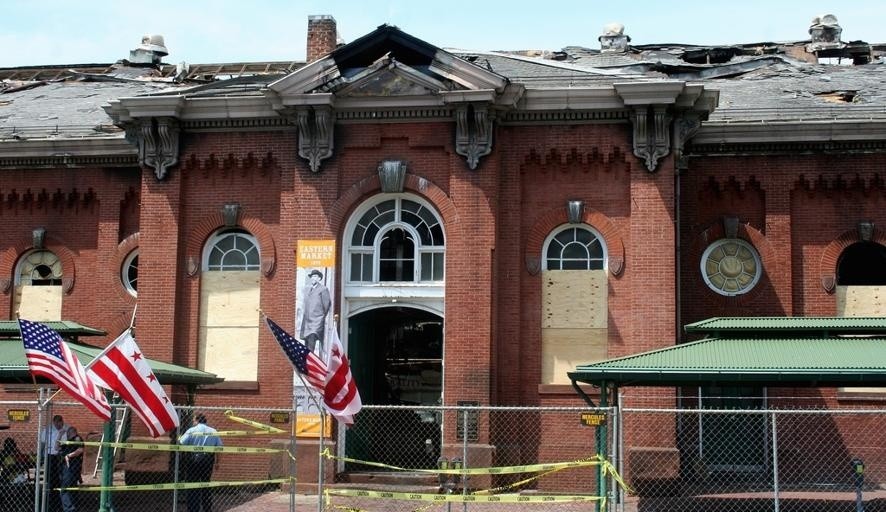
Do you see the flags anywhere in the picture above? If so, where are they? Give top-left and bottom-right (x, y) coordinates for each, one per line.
(265, 316), (329, 393)
(84, 330), (180, 438)
(328, 324), (361, 429)
(19, 317), (112, 421)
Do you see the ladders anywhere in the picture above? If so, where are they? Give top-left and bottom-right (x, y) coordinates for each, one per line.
(92, 302), (138, 478)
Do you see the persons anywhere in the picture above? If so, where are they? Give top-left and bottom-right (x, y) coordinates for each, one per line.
(0, 438), (21, 479)
(177, 412), (224, 511)
(53, 426), (85, 511)
(38, 415), (70, 511)
(299, 270), (331, 353)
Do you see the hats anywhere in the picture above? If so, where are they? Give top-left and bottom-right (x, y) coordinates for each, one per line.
(307, 270), (324, 281)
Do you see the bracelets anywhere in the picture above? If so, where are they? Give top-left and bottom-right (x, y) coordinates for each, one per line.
(66, 454), (70, 458)
